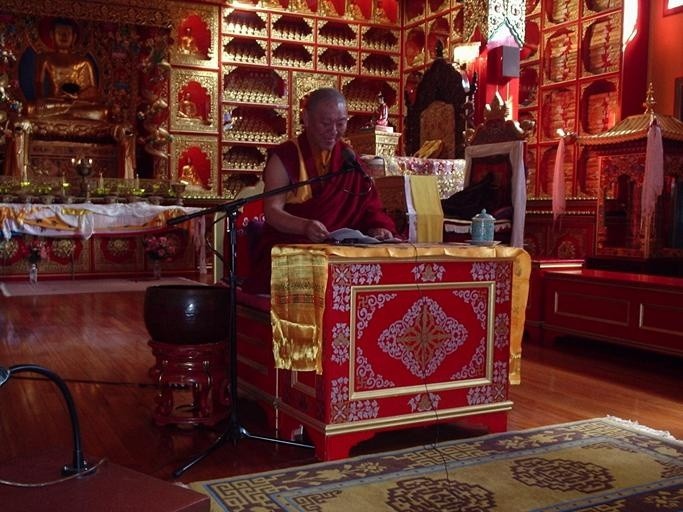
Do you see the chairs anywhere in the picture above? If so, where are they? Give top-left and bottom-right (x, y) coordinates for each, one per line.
(220, 196), (298, 424)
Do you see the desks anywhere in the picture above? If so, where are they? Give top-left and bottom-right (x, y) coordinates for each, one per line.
(275, 242), (515, 456)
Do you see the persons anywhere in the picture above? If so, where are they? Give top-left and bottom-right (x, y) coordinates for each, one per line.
(238, 87), (396, 298)
(177, 27), (200, 55)
(8, 16), (120, 143)
(178, 157), (206, 188)
(177, 92), (203, 121)
(362, 91), (391, 127)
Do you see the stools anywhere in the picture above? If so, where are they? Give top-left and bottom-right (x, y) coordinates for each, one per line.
(149, 344), (229, 423)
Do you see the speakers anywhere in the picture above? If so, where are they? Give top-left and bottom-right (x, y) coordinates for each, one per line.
(495, 45), (523, 80)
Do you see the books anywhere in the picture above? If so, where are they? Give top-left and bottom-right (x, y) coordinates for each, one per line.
(327, 226), (402, 244)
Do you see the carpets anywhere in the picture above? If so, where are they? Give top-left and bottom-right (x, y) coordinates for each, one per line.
(187, 414), (683, 512)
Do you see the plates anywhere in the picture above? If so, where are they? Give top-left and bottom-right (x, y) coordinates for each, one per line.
(464, 238), (502, 246)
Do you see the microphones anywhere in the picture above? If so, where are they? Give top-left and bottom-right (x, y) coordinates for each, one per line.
(343, 147), (371, 182)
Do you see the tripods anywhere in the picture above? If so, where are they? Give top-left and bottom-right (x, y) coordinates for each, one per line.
(166, 167), (350, 480)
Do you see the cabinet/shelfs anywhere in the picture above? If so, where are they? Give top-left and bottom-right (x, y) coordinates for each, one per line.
(162, 0), (650, 214)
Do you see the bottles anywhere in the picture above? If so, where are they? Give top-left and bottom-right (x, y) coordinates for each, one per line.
(471, 208), (497, 240)
(29, 262), (38, 284)
